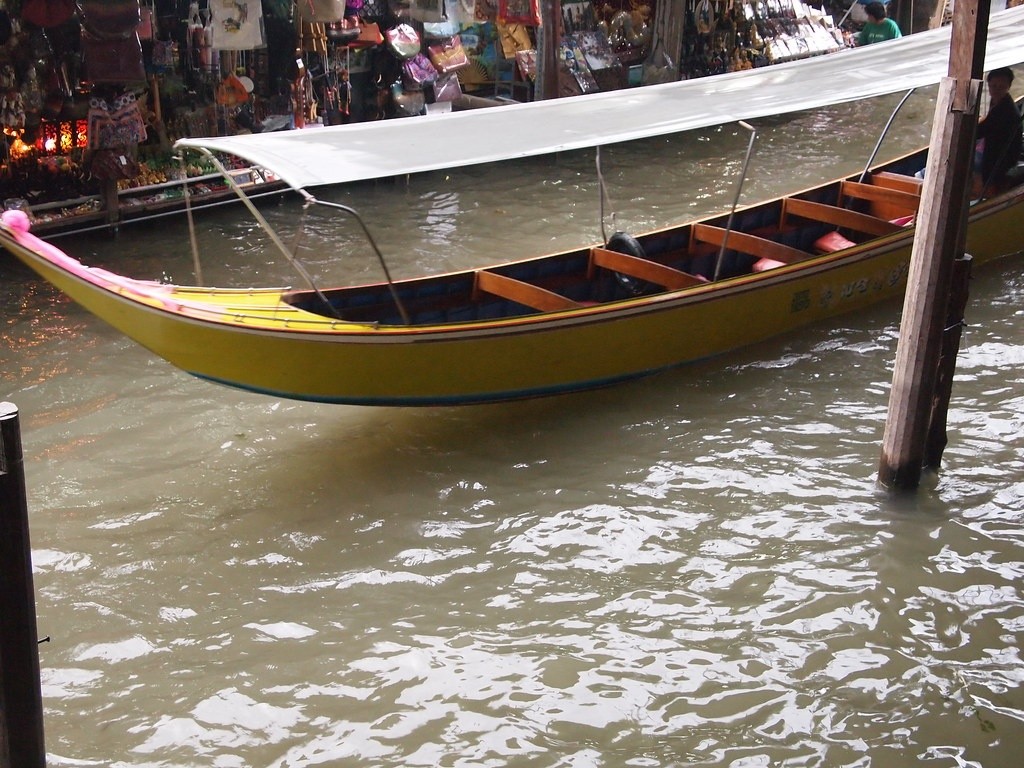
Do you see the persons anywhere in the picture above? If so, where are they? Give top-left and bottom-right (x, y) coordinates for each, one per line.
(977, 67), (1024, 196)
(856, 2), (903, 46)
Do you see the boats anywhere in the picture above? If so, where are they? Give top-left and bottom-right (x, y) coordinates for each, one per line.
(0, 3), (1024, 407)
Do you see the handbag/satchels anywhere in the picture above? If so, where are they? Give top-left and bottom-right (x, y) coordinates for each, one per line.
(19, 0), (148, 185)
(297, 0), (541, 116)
